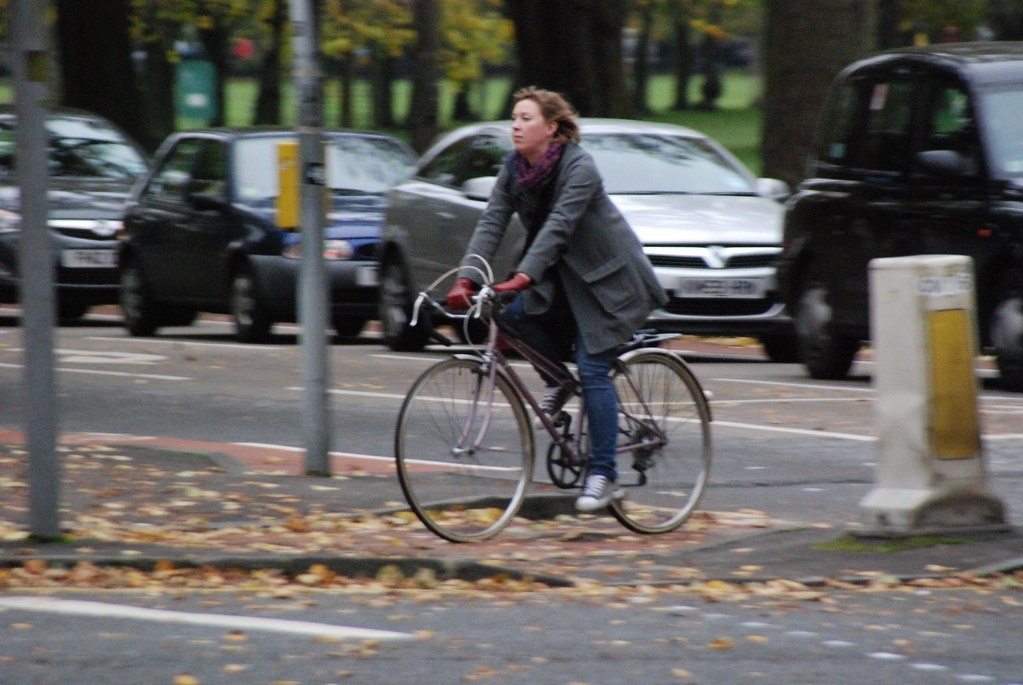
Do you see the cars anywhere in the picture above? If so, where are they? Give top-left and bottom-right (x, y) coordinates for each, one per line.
(374, 117), (800, 364)
(112, 124), (422, 340)
(1, 102), (152, 328)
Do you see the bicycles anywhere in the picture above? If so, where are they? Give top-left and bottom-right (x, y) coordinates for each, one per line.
(393, 252), (713, 541)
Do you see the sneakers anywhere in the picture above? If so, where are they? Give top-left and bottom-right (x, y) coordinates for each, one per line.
(574, 473), (624, 512)
(534, 379), (576, 430)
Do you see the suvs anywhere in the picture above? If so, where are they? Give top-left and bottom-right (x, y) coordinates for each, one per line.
(777, 32), (1022, 390)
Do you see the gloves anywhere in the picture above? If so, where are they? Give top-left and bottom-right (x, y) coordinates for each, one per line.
(491, 272), (531, 292)
(447, 278), (474, 310)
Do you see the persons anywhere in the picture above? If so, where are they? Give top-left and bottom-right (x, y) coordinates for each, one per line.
(449, 86), (664, 511)
